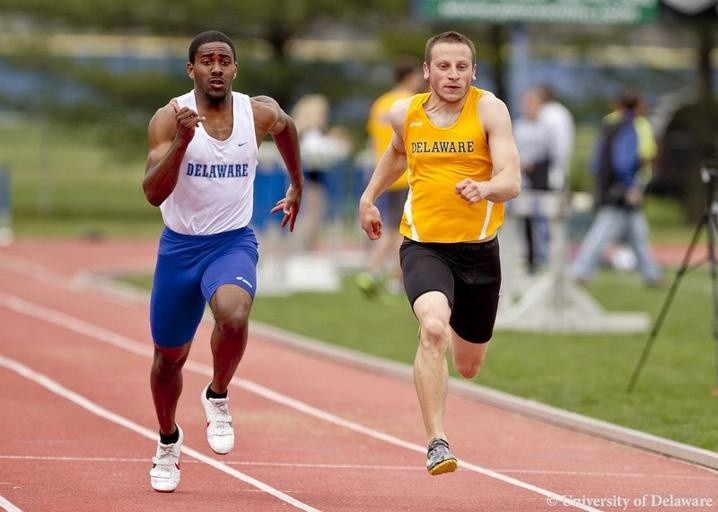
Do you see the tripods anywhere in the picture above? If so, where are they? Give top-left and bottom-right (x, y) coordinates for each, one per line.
(625, 193), (718, 395)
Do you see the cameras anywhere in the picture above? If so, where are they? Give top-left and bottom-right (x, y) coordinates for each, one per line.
(701, 166), (718, 193)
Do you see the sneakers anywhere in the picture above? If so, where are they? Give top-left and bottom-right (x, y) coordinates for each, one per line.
(150, 422), (184, 492)
(201, 380), (235, 453)
(426, 437), (458, 474)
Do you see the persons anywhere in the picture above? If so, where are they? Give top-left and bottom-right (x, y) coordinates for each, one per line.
(250, 56), (715, 302)
(140, 31), (304, 492)
(356, 30), (520, 476)
